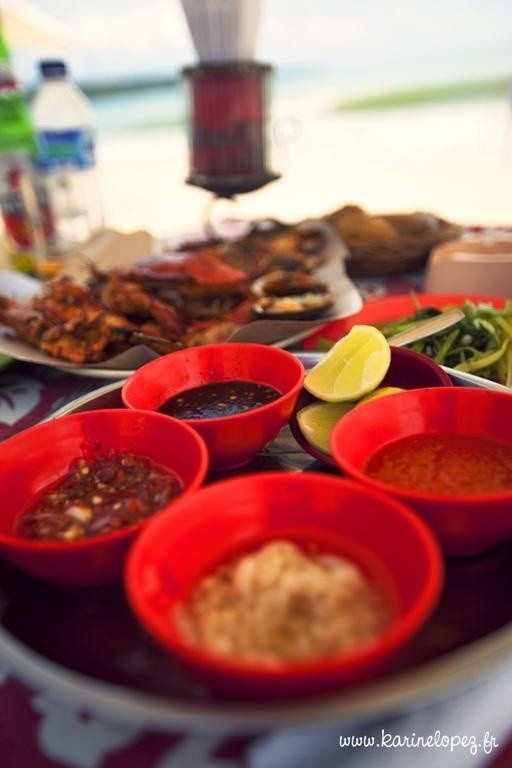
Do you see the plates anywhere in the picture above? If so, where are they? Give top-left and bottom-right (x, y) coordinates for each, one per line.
(281, 293), (509, 461)
(35, 252), (369, 377)
(2, 382), (510, 732)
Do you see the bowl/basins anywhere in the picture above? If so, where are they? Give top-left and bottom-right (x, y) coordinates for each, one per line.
(119, 337), (303, 472)
(119, 469), (441, 695)
(0, 408), (208, 590)
(330, 384), (512, 564)
(420, 232), (510, 305)
(288, 344), (453, 466)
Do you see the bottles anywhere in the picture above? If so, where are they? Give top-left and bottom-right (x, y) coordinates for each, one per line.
(25, 56), (105, 252)
(0, 24), (69, 278)
